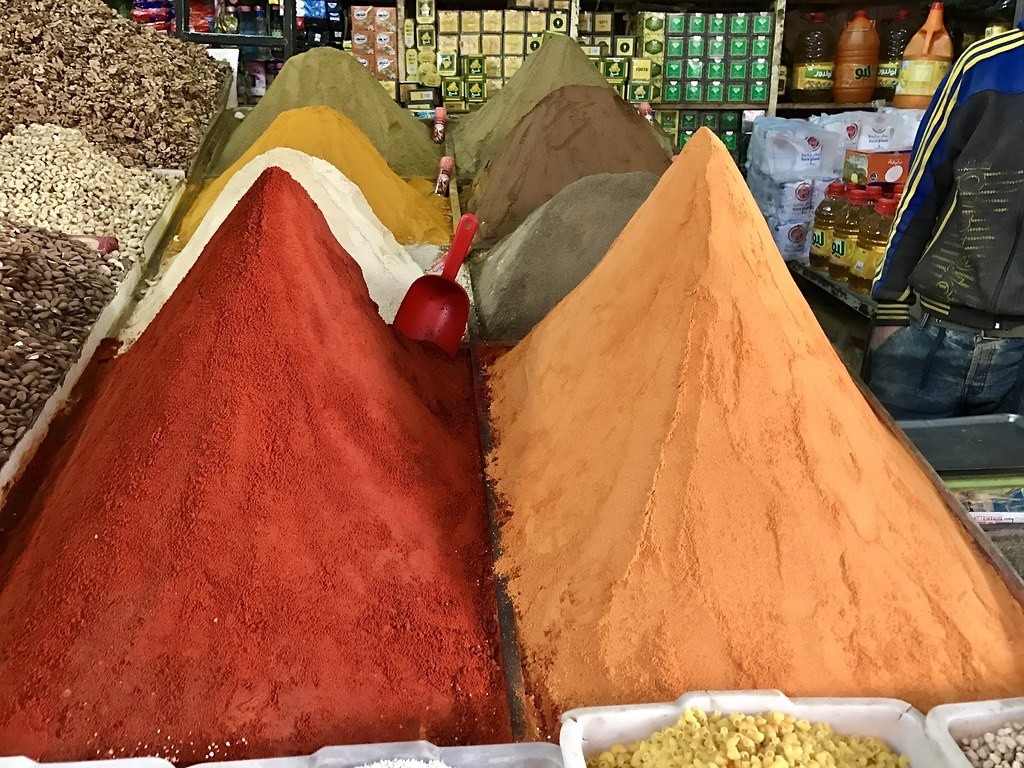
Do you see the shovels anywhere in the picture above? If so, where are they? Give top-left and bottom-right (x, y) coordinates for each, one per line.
(391, 212), (480, 359)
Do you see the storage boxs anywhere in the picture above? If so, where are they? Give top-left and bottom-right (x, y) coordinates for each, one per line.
(1, 690), (1024, 768)
(843, 149), (913, 187)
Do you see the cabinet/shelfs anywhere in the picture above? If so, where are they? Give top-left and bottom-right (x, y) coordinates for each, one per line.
(106, 0), (1023, 168)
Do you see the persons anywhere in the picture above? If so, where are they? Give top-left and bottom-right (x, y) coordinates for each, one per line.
(869, 18), (1024, 421)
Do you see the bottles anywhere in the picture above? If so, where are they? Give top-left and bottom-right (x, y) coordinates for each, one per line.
(808, 181), (905, 296)
(775, 0), (1018, 110)
(217, 5), (284, 106)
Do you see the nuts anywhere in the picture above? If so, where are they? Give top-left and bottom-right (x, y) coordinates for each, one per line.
(0, 122), (181, 472)
